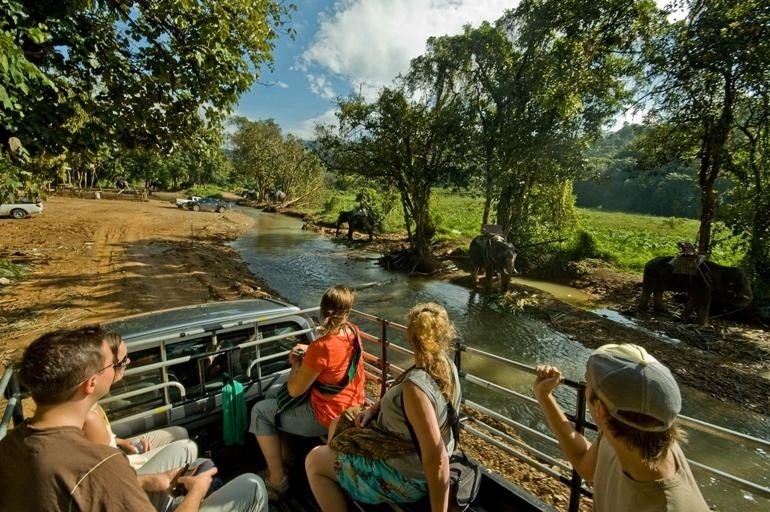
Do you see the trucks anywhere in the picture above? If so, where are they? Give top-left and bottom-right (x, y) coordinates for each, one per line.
(0, 295), (770, 512)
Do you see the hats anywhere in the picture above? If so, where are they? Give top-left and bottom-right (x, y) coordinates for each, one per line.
(584, 341), (683, 435)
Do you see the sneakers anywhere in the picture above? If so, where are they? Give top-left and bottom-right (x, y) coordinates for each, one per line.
(261, 474), (291, 502)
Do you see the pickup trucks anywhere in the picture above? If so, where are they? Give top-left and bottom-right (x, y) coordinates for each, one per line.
(0, 197), (43, 219)
(173, 195), (201, 212)
(187, 198), (231, 214)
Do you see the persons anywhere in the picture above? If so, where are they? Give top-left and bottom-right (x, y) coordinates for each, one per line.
(248, 285), (367, 495)
(304, 302), (465, 511)
(2, 328), (268, 511)
(532, 341), (714, 511)
(82, 326), (190, 511)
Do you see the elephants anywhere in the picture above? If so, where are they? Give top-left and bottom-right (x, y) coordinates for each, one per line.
(267, 189), (286, 205)
(637, 254), (755, 328)
(335, 209), (374, 241)
(469, 232), (520, 292)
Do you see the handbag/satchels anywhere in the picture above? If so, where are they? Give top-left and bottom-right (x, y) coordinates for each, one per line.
(446, 448), (482, 512)
(275, 350), (313, 412)
